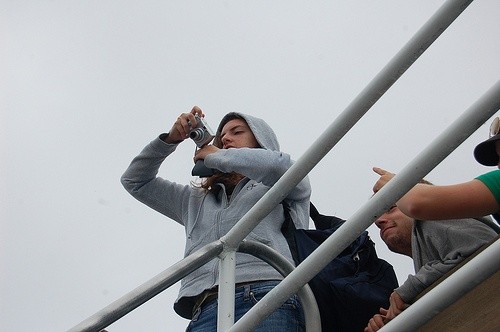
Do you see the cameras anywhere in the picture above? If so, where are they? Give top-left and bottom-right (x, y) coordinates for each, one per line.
(187, 114), (215, 148)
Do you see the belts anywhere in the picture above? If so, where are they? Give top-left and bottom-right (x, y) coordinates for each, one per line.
(192, 290), (217, 312)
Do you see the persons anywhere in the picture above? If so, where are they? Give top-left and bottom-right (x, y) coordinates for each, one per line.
(372, 118), (500, 224)
(120, 106), (312, 332)
(363, 178), (499, 332)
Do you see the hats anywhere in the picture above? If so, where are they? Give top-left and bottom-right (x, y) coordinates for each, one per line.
(474, 116), (500, 167)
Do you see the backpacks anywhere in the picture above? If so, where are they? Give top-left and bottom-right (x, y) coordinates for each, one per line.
(281, 197), (398, 332)
